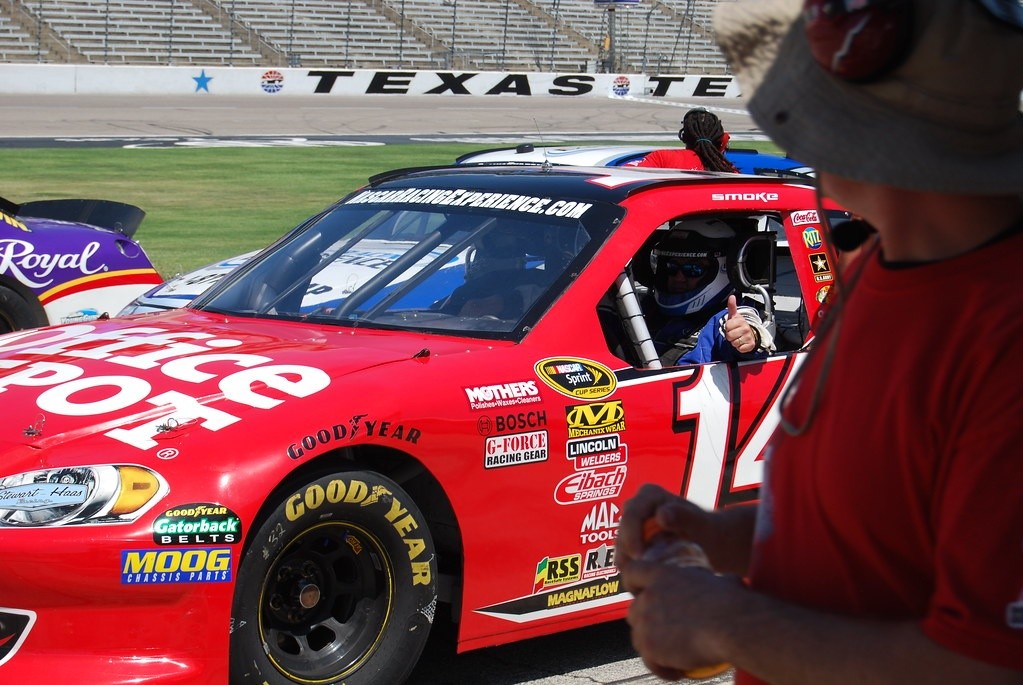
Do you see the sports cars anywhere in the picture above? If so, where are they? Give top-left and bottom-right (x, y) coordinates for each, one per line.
(0, 193), (162, 336)
(111, 134), (826, 337)
(1, 147), (866, 684)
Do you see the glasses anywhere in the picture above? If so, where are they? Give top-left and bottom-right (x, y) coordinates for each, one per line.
(662, 260), (712, 278)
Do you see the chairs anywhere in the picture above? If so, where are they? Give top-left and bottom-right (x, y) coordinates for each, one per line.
(725, 217), (779, 343)
(430, 226), (547, 320)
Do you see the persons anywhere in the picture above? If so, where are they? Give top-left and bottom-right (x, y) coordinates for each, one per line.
(637, 109), (747, 176)
(615, 1), (1023, 685)
(460, 216), (790, 370)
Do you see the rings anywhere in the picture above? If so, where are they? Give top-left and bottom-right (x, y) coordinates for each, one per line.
(738, 338), (743, 345)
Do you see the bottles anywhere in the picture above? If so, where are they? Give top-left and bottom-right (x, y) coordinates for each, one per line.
(641, 518), (731, 680)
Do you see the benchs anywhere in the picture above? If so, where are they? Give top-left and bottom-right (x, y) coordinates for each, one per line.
(0, 0), (736, 75)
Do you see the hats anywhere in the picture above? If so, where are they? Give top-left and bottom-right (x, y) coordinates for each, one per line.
(744, 2), (1022, 196)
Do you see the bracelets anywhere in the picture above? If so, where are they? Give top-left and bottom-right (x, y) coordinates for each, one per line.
(750, 326), (760, 342)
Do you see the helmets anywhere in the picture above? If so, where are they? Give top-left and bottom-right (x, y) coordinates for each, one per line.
(650, 217), (737, 320)
